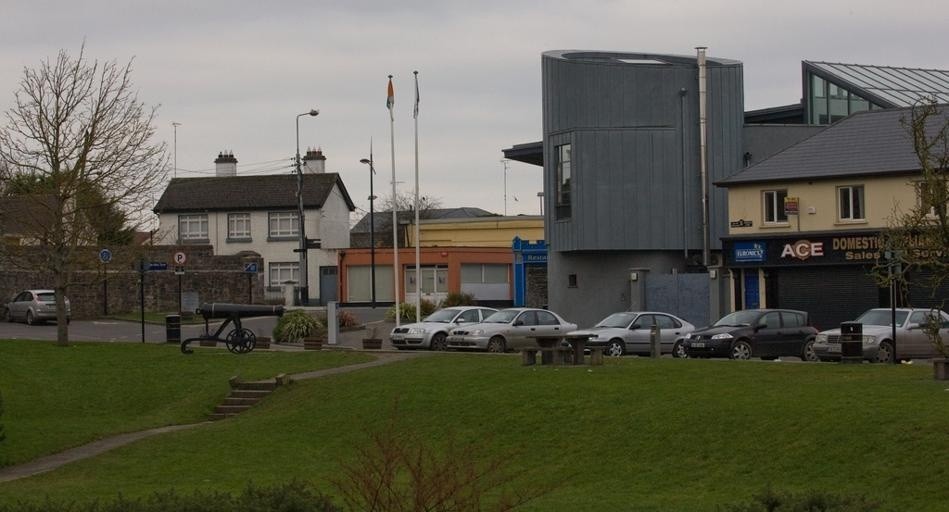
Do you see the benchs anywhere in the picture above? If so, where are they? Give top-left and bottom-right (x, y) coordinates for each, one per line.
(516, 347), (570, 367)
(555, 345), (610, 365)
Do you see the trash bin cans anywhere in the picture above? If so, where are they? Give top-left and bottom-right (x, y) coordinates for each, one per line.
(165, 315), (181, 344)
(840, 323), (862, 364)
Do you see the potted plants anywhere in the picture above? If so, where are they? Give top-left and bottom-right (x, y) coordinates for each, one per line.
(199, 327), (223, 347)
(304, 326), (324, 350)
(362, 329), (382, 349)
(917, 308), (949, 380)
(252, 329), (273, 348)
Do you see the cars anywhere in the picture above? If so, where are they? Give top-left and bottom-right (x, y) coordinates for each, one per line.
(4, 289), (71, 325)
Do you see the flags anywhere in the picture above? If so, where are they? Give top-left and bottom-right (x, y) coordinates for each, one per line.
(412, 77), (420, 120)
(385, 78), (395, 121)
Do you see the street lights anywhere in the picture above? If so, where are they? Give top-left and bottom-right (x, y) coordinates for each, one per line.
(296, 109), (319, 306)
(360, 137), (378, 308)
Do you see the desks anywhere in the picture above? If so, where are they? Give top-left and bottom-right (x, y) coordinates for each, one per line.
(527, 335), (599, 366)
